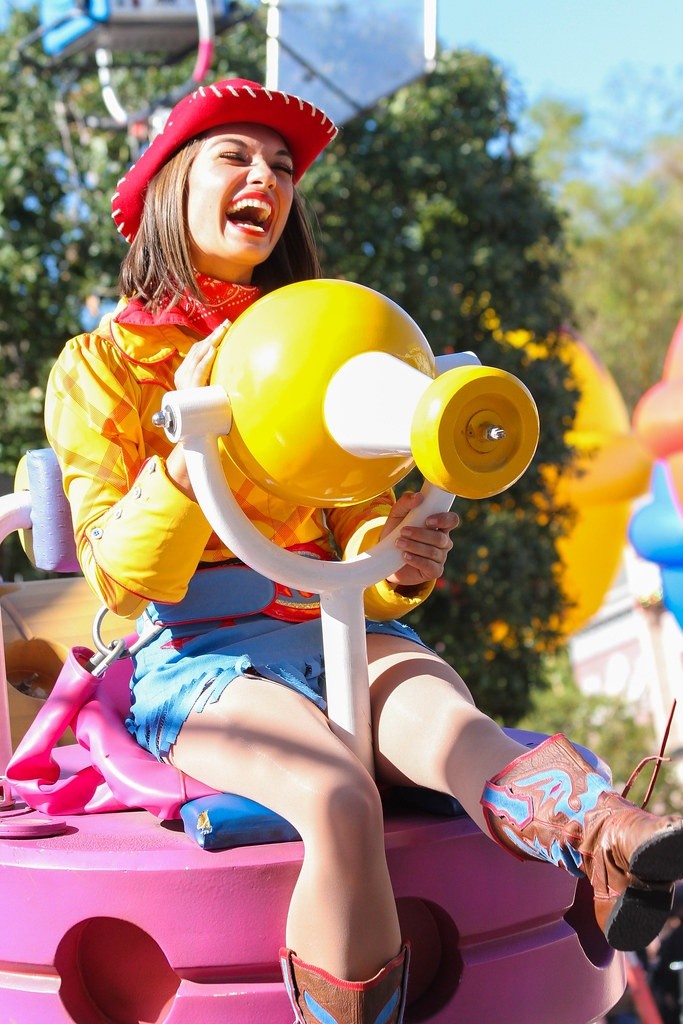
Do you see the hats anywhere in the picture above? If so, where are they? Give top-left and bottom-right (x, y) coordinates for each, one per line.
(111, 78), (339, 244)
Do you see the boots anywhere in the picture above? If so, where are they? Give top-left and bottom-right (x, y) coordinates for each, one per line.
(280, 941), (411, 1024)
(477, 699), (683, 950)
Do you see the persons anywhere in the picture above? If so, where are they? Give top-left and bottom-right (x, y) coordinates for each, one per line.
(44, 81), (682, 1024)
